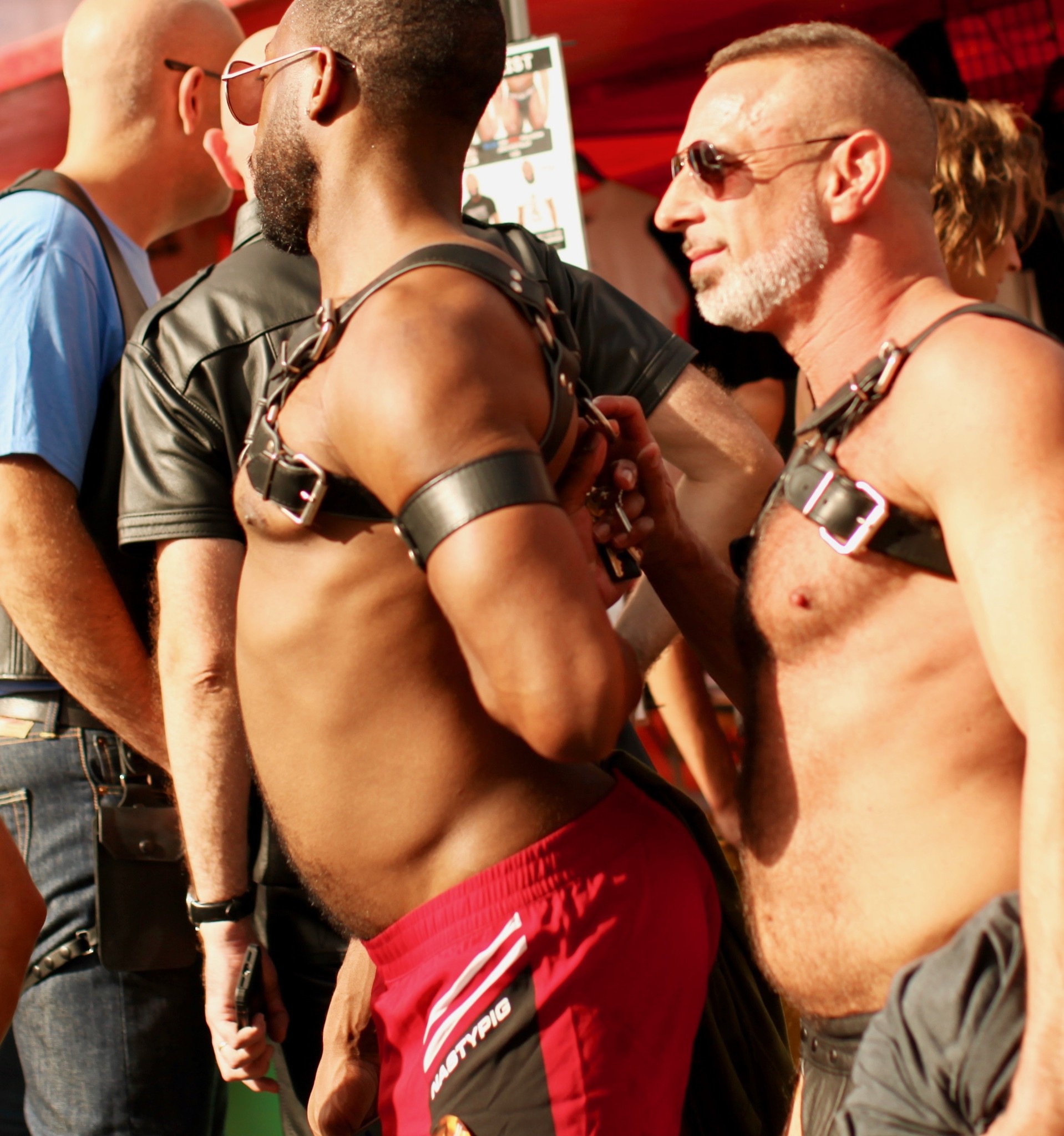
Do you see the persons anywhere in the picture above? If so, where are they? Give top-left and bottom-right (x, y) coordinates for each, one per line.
(0, 0), (1064, 1136)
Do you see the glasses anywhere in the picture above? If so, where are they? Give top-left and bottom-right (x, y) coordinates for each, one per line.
(221, 46), (355, 126)
(670, 135), (851, 186)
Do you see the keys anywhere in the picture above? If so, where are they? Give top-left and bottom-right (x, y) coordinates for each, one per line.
(586, 480), (642, 577)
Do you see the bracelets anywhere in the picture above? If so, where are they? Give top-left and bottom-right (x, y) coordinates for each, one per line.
(186, 878), (258, 931)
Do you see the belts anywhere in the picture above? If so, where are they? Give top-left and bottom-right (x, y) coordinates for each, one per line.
(0, 696), (108, 730)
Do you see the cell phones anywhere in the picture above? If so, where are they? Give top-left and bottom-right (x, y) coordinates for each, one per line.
(234, 943), (271, 1044)
